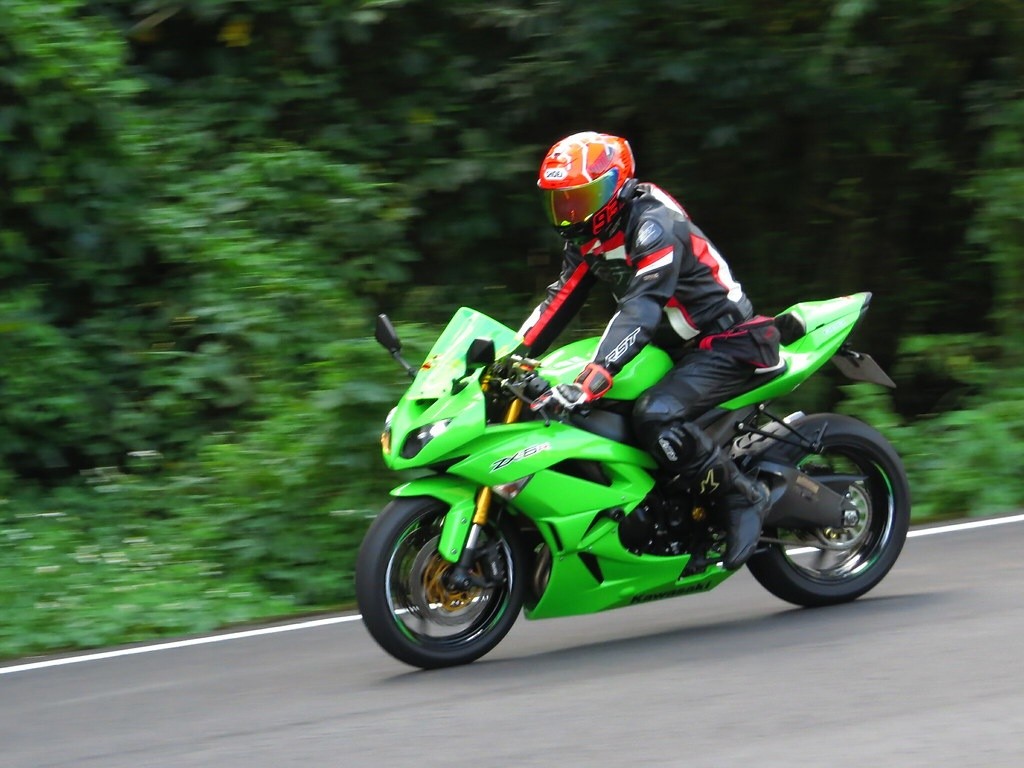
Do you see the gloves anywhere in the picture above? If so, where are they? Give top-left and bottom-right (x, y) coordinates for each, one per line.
(530, 361), (611, 420)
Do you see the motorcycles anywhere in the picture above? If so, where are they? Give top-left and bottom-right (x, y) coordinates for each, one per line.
(355, 288), (912, 671)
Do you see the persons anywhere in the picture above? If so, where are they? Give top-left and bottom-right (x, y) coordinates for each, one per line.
(481, 131), (781, 571)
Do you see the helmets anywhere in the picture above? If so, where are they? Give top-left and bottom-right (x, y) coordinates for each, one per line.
(537, 130), (635, 242)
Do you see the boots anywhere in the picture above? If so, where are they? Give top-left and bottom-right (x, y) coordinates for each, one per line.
(683, 443), (773, 571)
